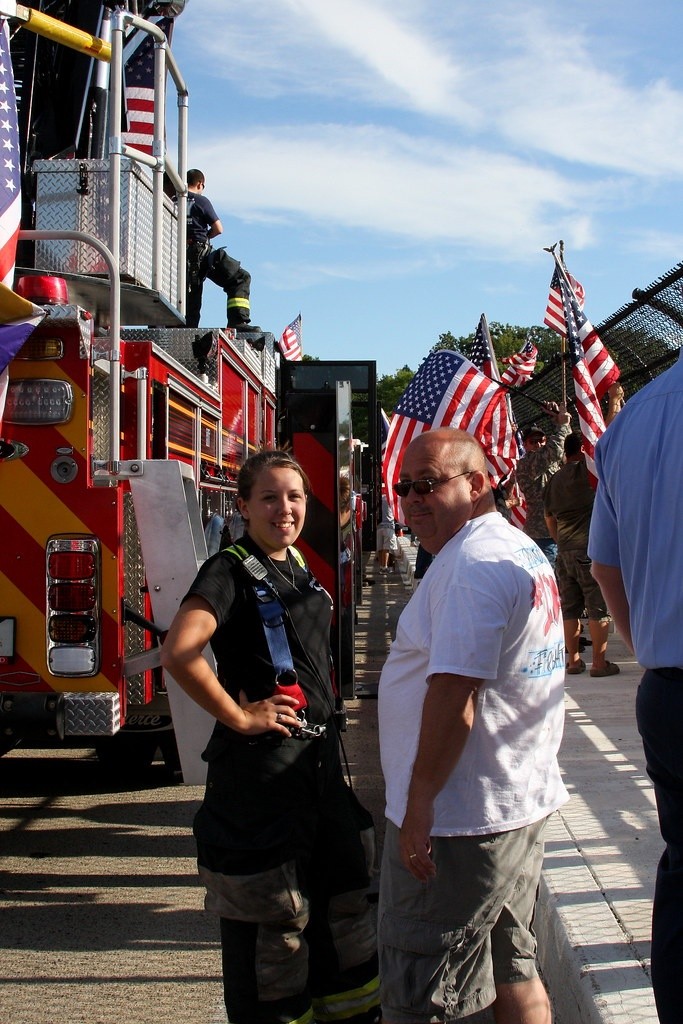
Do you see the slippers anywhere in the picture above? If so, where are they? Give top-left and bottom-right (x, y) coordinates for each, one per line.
(565, 658), (586, 674)
(590, 659), (620, 676)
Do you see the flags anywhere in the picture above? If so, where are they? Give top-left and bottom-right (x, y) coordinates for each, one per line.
(378, 238), (621, 536)
(1, 15), (21, 290)
(115, 14), (176, 163)
(278, 312), (303, 361)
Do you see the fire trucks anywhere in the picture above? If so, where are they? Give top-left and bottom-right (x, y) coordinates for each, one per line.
(1, 0), (382, 794)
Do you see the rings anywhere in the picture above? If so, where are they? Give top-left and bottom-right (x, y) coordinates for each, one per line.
(409, 853), (417, 859)
(276, 714), (282, 721)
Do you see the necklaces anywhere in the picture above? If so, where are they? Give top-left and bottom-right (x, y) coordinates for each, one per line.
(250, 533), (301, 592)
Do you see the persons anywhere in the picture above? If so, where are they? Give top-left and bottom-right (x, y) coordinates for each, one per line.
(489, 399), (590, 576)
(587, 343), (683, 1024)
(182, 168), (263, 332)
(370, 423), (570, 1023)
(339, 465), (351, 683)
(542, 382), (626, 678)
(358, 478), (437, 592)
(159, 450), (386, 1023)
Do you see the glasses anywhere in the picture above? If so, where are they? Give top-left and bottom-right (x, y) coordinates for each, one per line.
(526, 437), (546, 444)
(393, 470), (475, 497)
(198, 180), (205, 190)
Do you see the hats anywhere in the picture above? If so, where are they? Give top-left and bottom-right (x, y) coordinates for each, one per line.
(522, 427), (546, 442)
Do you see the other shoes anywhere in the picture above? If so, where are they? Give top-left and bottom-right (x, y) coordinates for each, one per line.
(232, 323), (262, 333)
(363, 579), (375, 586)
(379, 567), (395, 574)
